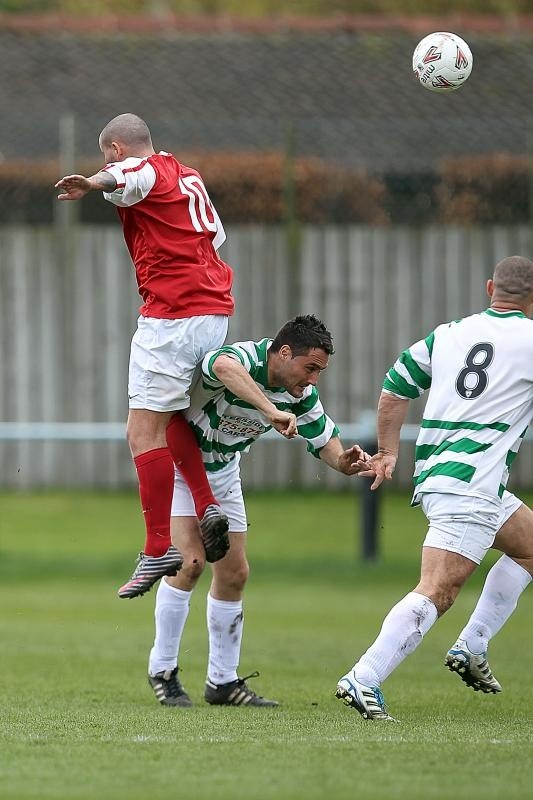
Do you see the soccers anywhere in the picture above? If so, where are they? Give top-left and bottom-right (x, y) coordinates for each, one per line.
(411, 30), (474, 94)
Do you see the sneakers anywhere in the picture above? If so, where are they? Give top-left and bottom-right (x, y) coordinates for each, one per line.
(148, 667), (192, 708)
(204, 670), (279, 706)
(116, 543), (184, 600)
(198, 503), (231, 563)
(334, 670), (394, 720)
(444, 637), (503, 694)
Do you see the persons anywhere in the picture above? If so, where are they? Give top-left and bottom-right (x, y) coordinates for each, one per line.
(334, 255), (533, 722)
(147, 316), (374, 705)
(54, 111), (238, 599)
